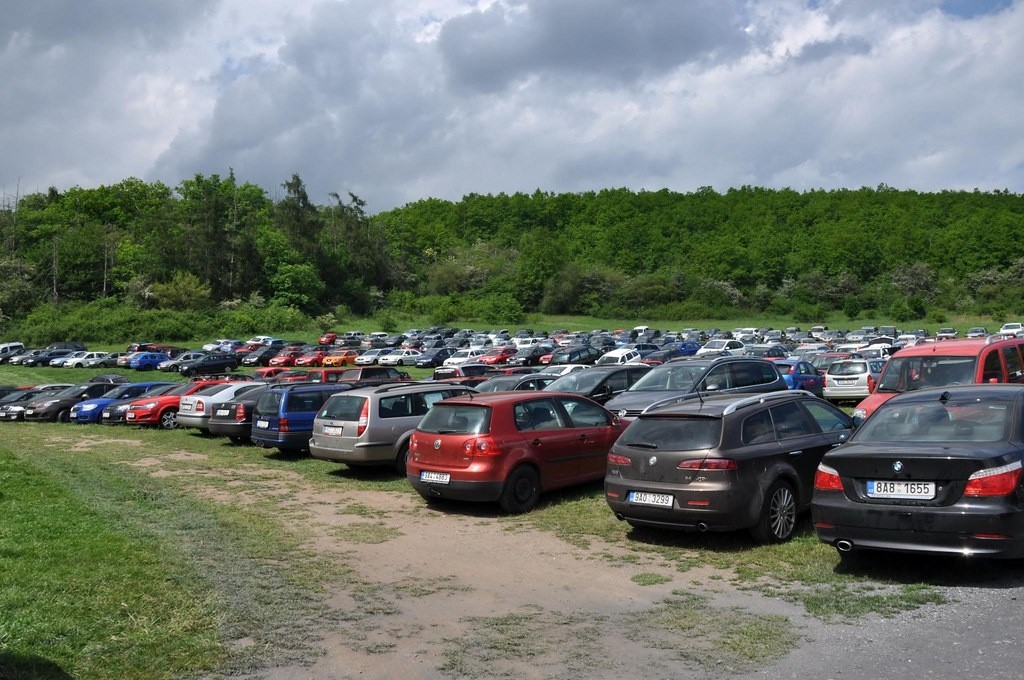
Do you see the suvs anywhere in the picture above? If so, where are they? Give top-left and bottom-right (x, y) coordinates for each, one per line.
(309, 380), (483, 477)
(602, 388), (858, 546)
(250, 380), (367, 455)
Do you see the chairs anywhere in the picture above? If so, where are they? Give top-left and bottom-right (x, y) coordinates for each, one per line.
(452, 415), (468, 433)
(918, 404), (952, 428)
(391, 401), (406, 416)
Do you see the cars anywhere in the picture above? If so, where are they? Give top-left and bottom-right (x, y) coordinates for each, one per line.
(207, 385), (276, 445)
(0, 320), (1024, 433)
(809, 380), (1024, 561)
(405, 390), (630, 516)
